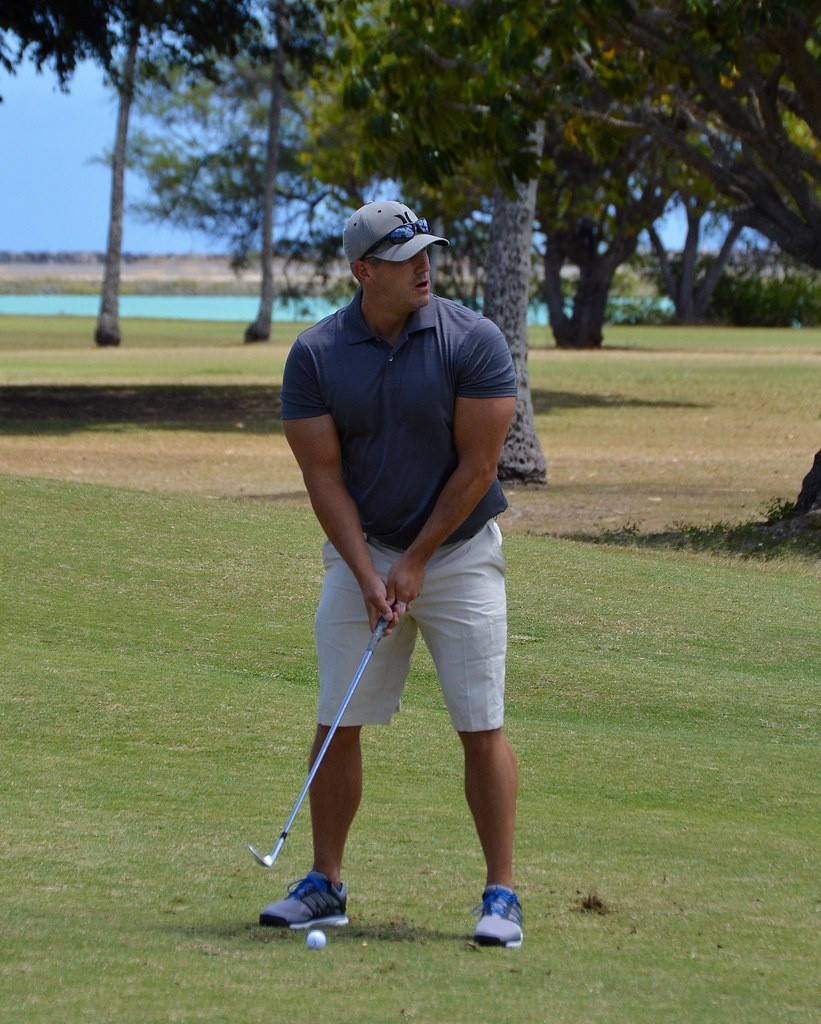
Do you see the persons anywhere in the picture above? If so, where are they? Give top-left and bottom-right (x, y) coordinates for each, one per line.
(261, 202), (525, 946)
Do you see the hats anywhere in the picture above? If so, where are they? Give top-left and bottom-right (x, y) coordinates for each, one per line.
(342, 200), (450, 262)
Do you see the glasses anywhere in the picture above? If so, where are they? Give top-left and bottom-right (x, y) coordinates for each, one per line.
(359, 217), (432, 261)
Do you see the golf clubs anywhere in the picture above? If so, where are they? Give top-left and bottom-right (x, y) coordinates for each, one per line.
(247, 608), (390, 868)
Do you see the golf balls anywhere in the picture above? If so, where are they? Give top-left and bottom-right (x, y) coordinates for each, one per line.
(307, 930), (327, 950)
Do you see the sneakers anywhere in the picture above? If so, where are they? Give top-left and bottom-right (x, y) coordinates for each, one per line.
(471, 884), (523, 948)
(259, 873), (349, 929)
